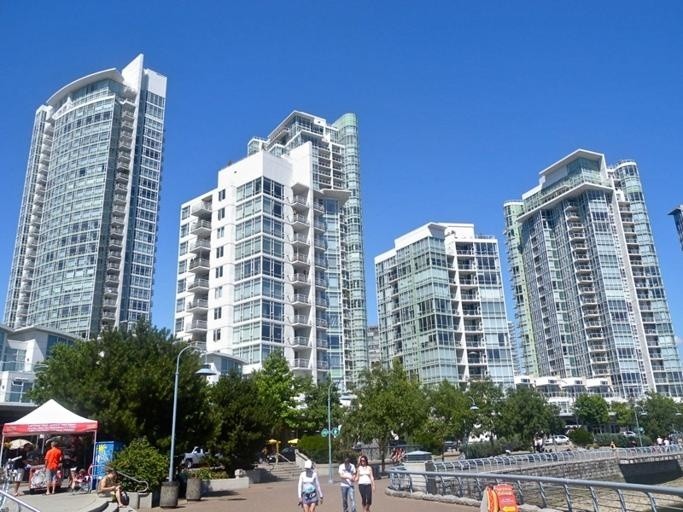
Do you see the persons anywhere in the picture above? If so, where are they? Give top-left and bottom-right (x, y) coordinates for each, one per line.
(397, 447), (406, 463)
(70, 466), (86, 489)
(337, 457), (356, 511)
(656, 435), (663, 446)
(66, 444), (78, 487)
(43, 441), (63, 495)
(352, 455), (376, 511)
(96, 471), (126, 508)
(663, 436), (670, 452)
(296, 459), (324, 512)
(11, 442), (33, 496)
(391, 447), (400, 463)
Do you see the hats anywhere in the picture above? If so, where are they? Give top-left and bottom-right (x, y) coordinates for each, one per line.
(304, 459), (312, 469)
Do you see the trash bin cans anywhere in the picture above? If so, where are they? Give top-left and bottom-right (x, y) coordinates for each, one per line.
(159, 481), (180, 507)
(185, 478), (202, 501)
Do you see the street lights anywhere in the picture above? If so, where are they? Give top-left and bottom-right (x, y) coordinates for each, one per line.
(328, 380), (351, 484)
(169, 345), (217, 481)
(633, 406), (647, 447)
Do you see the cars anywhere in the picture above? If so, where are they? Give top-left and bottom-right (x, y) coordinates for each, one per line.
(544, 435), (569, 446)
(619, 431), (635, 437)
(180, 445), (210, 469)
